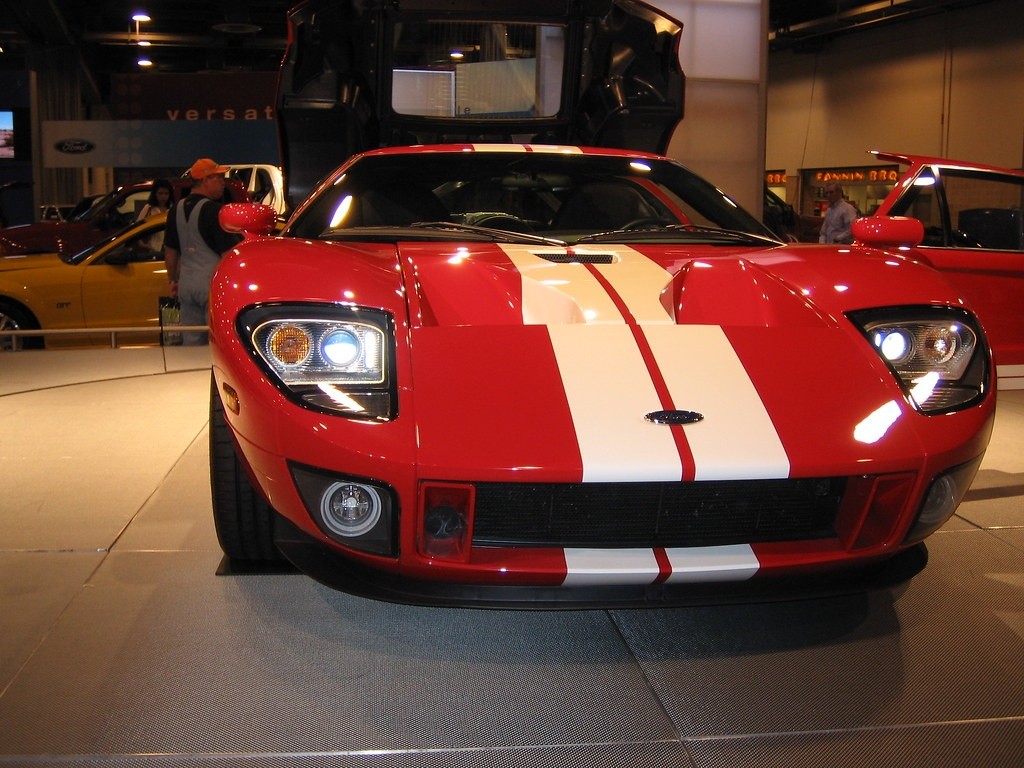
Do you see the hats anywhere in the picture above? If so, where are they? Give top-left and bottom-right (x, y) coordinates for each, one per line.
(190, 158), (231, 179)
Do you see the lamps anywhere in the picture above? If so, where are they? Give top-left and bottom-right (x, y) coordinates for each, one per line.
(137, 41), (151, 46)
(129, 7), (152, 21)
(137, 50), (156, 66)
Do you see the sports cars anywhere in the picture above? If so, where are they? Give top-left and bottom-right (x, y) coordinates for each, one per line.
(207, 140), (996, 613)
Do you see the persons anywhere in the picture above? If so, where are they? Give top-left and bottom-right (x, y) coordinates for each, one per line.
(817, 181), (861, 245)
(163, 157), (244, 346)
(133, 178), (175, 253)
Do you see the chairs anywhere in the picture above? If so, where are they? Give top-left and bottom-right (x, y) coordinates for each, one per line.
(360, 171), (437, 227)
(548, 162), (659, 234)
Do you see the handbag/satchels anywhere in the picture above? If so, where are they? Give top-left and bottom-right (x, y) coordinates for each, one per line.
(161, 298), (182, 345)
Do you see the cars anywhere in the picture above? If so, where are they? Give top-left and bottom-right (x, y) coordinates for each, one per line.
(1, 162), (290, 352)
(848, 149), (1024, 390)
